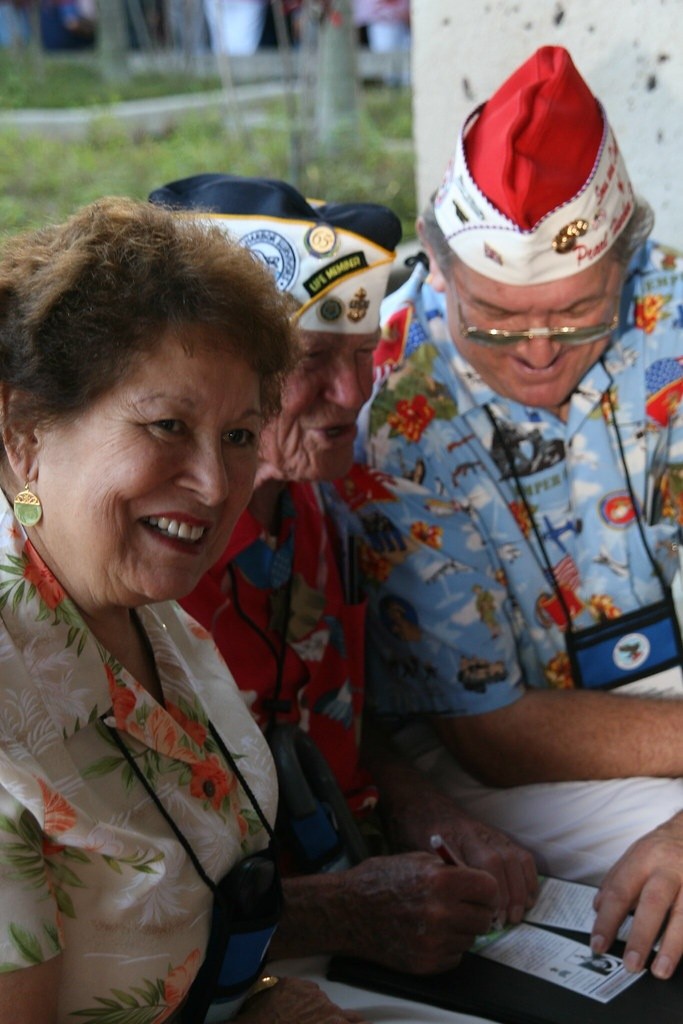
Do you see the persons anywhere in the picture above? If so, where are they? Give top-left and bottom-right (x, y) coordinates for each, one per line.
(322, 45), (683, 979)
(151, 175), (542, 973)
(2, 195), (357, 1024)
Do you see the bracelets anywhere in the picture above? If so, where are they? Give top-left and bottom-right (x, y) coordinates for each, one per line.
(248, 977), (279, 996)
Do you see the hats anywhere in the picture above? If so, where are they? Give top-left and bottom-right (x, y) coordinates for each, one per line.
(148, 175), (404, 334)
(432, 45), (637, 285)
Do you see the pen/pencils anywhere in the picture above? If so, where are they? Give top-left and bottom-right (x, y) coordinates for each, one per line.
(429, 833), (458, 863)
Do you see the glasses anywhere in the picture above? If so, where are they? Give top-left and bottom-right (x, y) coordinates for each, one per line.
(448, 265), (624, 350)
